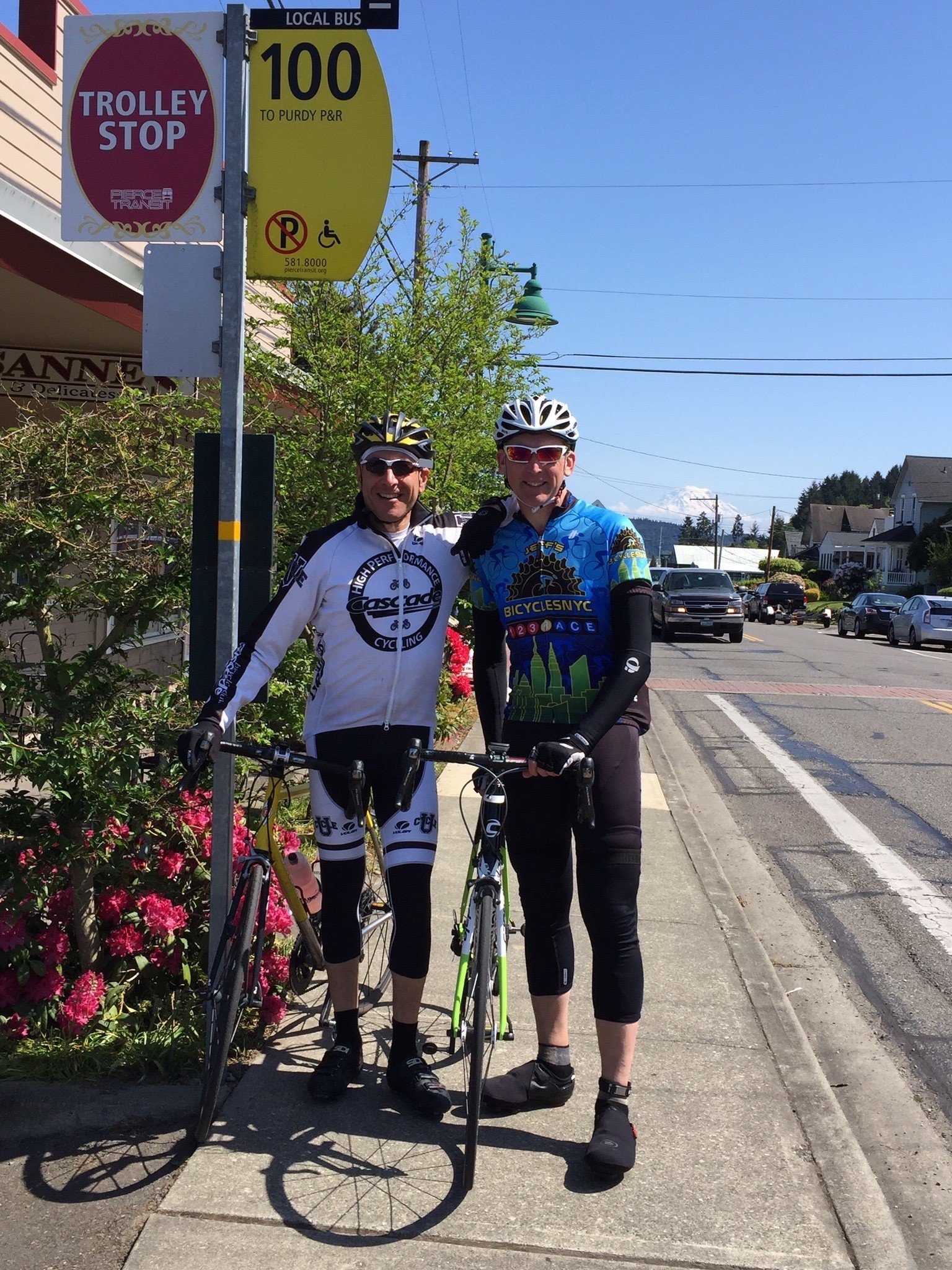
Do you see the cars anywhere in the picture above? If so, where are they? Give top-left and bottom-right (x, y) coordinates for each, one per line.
(888, 594), (952, 650)
(736, 589), (754, 618)
(838, 593), (909, 639)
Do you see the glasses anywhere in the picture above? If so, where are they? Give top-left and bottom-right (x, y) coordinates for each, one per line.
(360, 457), (419, 477)
(503, 445), (569, 463)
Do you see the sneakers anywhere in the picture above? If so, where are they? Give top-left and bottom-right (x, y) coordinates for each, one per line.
(386, 1050), (451, 1114)
(585, 1104), (637, 1174)
(481, 1059), (575, 1113)
(307, 1044), (363, 1098)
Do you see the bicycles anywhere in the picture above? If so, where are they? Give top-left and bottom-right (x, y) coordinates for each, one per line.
(415, 738), (597, 1192)
(177, 738), (396, 1144)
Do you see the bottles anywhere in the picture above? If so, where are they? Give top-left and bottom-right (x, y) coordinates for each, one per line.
(284, 852), (322, 914)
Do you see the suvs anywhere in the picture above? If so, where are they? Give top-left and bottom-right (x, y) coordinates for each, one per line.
(747, 582), (808, 625)
(649, 567), (747, 643)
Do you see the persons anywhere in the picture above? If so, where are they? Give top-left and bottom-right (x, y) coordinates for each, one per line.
(453, 396), (654, 1173)
(173, 407), (523, 1113)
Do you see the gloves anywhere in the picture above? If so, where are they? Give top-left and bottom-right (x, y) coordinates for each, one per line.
(451, 507), (504, 567)
(177, 720), (222, 773)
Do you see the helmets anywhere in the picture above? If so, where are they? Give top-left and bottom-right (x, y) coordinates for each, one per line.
(493, 395), (579, 444)
(350, 410), (435, 469)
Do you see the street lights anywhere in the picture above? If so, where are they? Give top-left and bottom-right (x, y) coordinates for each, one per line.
(474, 232), (559, 424)
